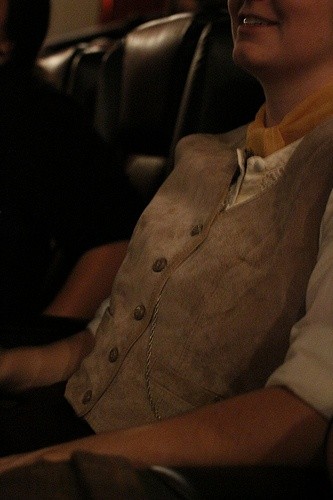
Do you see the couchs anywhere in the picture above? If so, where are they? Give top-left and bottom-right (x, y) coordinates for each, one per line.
(40, 10), (217, 201)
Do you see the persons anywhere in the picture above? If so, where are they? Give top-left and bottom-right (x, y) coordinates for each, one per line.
(0, 0), (333, 500)
(0, 0), (134, 410)
